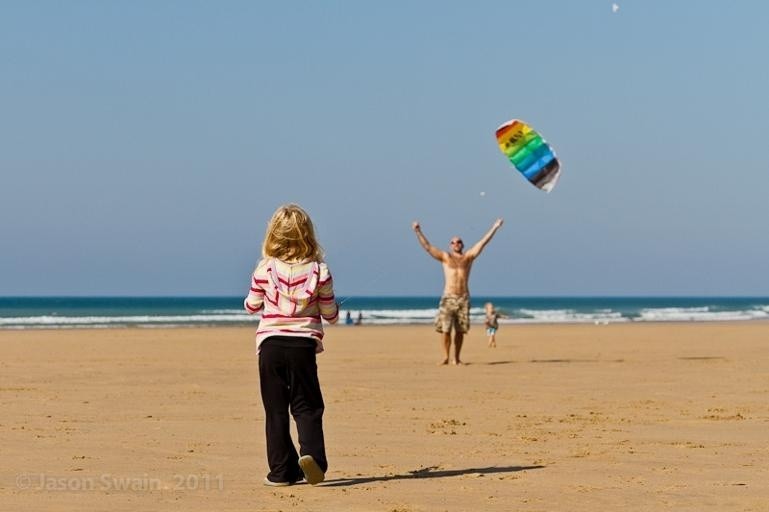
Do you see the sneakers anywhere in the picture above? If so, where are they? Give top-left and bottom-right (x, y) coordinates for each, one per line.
(265, 454), (324, 488)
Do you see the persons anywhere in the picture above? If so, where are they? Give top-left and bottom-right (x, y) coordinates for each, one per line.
(483, 302), (509, 347)
(346, 311), (353, 324)
(243, 203), (340, 486)
(412, 219), (504, 367)
(355, 312), (363, 325)
